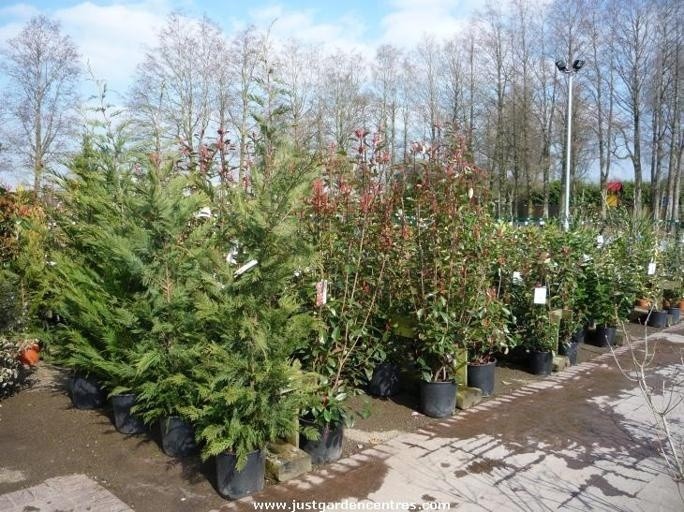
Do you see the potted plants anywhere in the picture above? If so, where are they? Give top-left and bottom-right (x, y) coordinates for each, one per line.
(0, 58), (684, 500)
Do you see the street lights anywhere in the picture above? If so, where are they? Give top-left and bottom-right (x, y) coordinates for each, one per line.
(555, 55), (587, 234)
